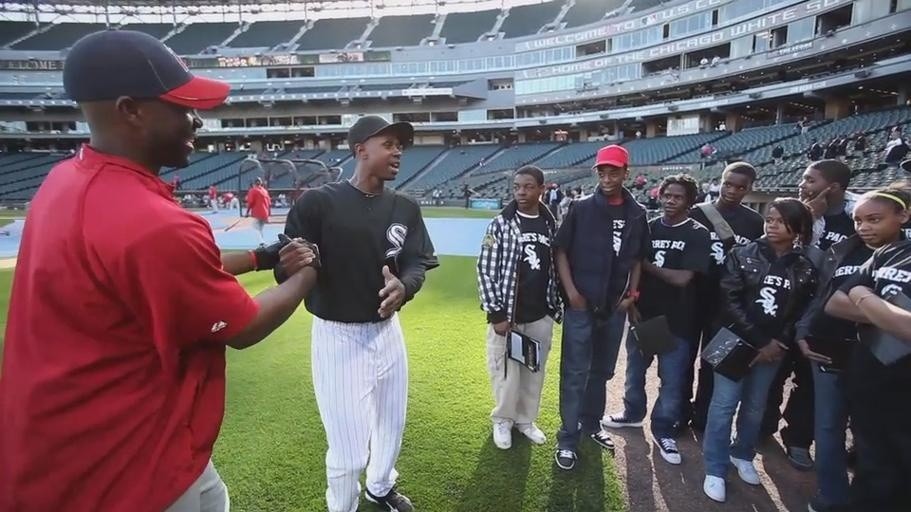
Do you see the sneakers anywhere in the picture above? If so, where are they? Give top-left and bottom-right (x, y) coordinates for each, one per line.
(523, 423), (546, 445)
(554, 450), (577, 470)
(601, 413), (642, 428)
(729, 455), (760, 486)
(493, 423), (511, 450)
(780, 430), (814, 471)
(703, 475), (726, 503)
(590, 430), (615, 450)
(652, 434), (681, 465)
(364, 488), (413, 512)
(807, 489), (833, 512)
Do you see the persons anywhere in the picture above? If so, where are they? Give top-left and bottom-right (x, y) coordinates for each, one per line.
(432, 183), (445, 206)
(245, 176), (271, 244)
(279, 191), (288, 206)
(266, 113), (440, 511)
(476, 94), (911, 511)
(463, 182), (475, 209)
(168, 173), (241, 214)
(1, 28), (320, 512)
(460, 149), (467, 154)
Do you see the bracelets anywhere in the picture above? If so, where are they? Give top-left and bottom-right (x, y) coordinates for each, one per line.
(248, 250), (259, 272)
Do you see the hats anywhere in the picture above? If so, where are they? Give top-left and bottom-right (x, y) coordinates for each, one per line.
(62, 28), (230, 109)
(591, 144), (628, 169)
(347, 115), (413, 153)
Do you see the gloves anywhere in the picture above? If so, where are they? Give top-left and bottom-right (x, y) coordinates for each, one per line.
(278, 233), (324, 281)
(254, 242), (284, 272)
